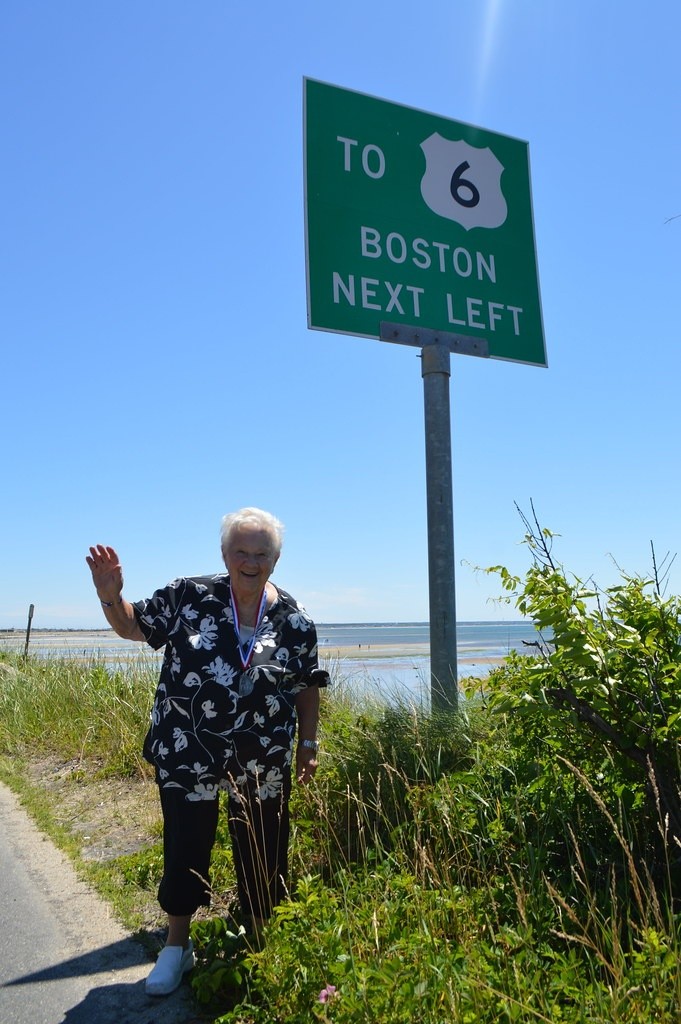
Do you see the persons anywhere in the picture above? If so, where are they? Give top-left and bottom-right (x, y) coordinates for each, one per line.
(85, 508), (331, 994)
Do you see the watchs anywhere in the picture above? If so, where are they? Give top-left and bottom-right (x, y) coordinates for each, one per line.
(297, 738), (319, 752)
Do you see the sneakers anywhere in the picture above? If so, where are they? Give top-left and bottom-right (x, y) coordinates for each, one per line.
(145, 937), (195, 996)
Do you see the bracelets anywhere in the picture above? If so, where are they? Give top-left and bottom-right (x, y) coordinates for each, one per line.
(100, 594), (121, 607)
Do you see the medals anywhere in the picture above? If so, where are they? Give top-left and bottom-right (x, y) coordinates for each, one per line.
(238, 675), (253, 696)
